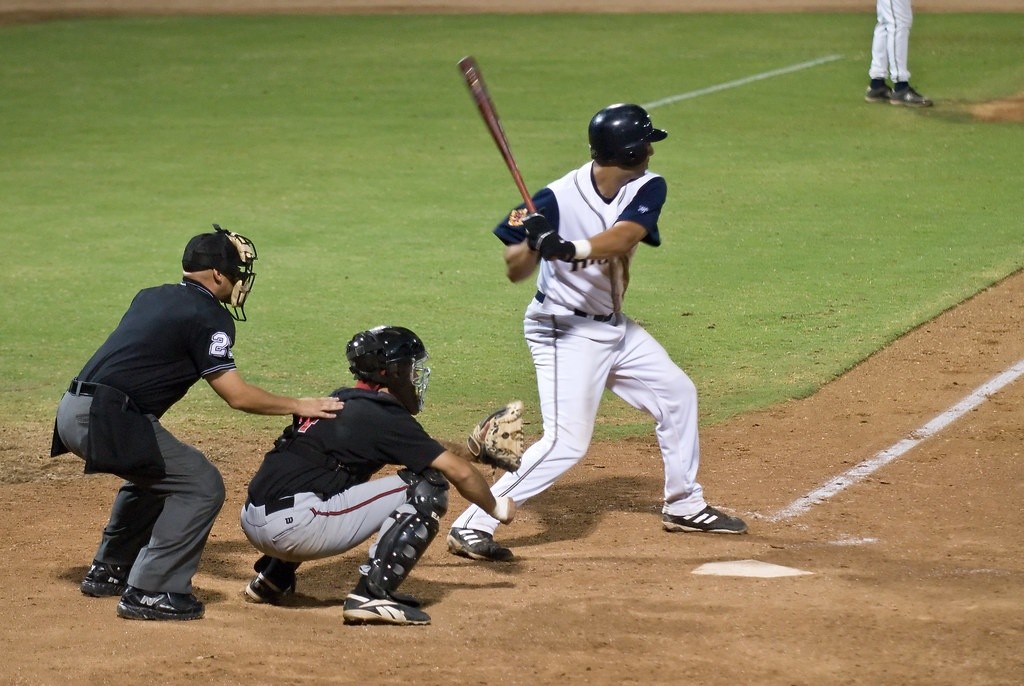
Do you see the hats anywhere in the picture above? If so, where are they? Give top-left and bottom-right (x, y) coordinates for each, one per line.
(182, 232), (248, 271)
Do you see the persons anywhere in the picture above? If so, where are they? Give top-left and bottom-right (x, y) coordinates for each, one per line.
(50, 224), (345, 621)
(865, 0), (932, 108)
(446, 102), (748, 560)
(253, 326), (516, 625)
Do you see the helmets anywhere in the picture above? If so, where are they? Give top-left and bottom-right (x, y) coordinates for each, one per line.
(588, 103), (669, 167)
(346, 325), (429, 414)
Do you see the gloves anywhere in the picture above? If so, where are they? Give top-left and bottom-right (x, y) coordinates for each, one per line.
(522, 214), (577, 262)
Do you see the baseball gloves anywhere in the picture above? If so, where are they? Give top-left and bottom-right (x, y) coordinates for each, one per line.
(466, 399), (525, 474)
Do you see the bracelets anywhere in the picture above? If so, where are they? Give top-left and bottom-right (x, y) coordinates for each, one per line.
(569, 240), (591, 260)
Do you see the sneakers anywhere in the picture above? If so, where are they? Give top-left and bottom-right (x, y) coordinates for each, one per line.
(865, 86), (895, 102)
(115, 586), (207, 619)
(446, 525), (513, 562)
(79, 558), (135, 598)
(889, 87), (934, 108)
(247, 573), (292, 604)
(342, 591), (431, 626)
(661, 503), (748, 535)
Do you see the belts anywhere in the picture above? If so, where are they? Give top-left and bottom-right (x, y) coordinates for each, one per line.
(244, 494), (297, 516)
(534, 290), (615, 322)
(68, 380), (96, 397)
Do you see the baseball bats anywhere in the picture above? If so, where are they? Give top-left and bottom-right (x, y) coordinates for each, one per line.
(455, 54), (559, 261)
